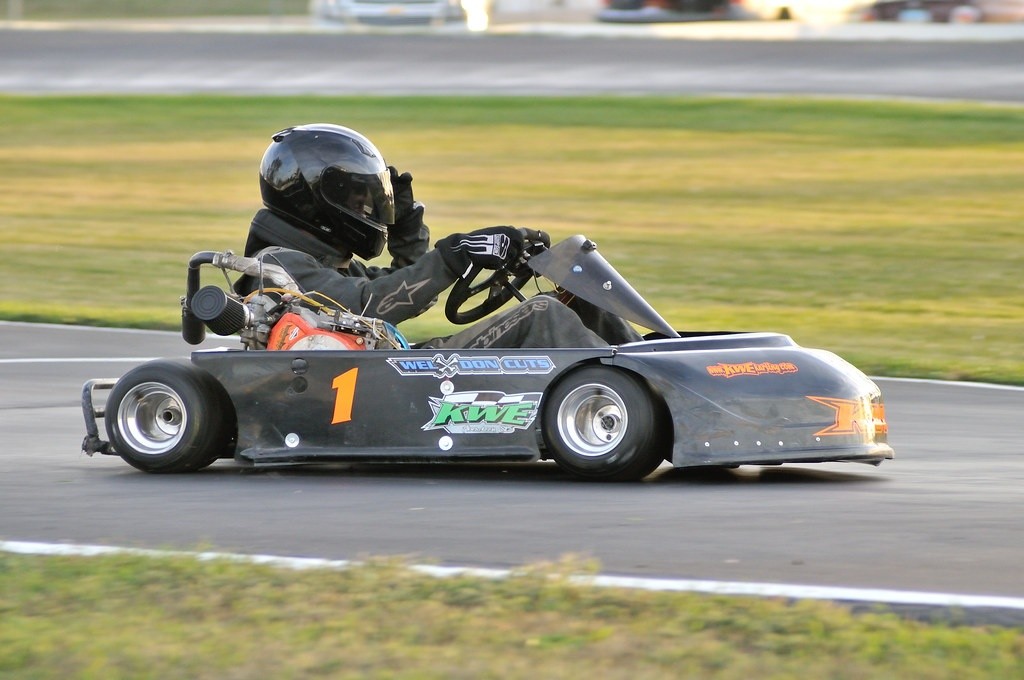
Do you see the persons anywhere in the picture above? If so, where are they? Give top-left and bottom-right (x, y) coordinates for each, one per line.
(231, 123), (644, 348)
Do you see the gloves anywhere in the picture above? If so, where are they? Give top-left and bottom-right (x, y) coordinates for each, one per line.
(435, 225), (525, 280)
(390, 162), (425, 241)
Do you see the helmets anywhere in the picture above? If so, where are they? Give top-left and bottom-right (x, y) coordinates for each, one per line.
(259, 123), (392, 258)
